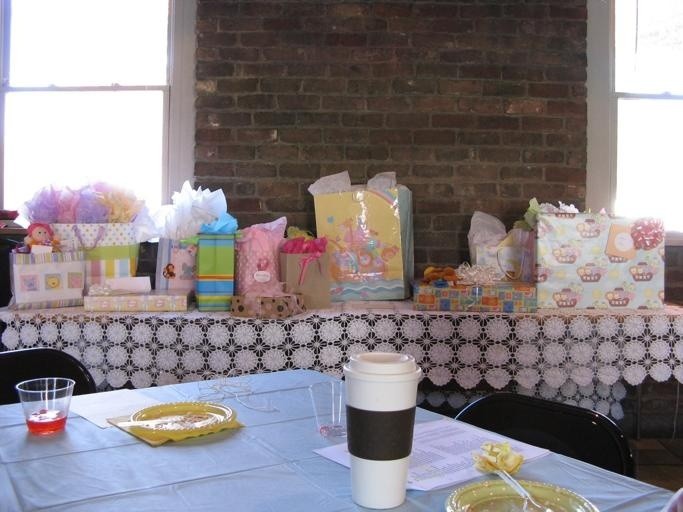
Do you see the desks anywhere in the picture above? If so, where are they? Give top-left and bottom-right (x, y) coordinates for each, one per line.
(0, 298), (683, 440)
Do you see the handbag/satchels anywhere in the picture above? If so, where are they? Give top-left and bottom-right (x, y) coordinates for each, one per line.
(468, 241), (534, 283)
(51, 220), (142, 298)
(155, 239), (197, 292)
(187, 204), (242, 314)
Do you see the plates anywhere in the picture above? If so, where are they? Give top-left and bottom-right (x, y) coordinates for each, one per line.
(132, 401), (237, 440)
(445, 479), (597, 511)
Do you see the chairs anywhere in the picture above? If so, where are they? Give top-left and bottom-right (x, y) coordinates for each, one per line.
(0, 346), (98, 406)
(454, 390), (638, 481)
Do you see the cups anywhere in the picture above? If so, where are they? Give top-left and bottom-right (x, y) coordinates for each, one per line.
(342, 351), (423, 507)
(16, 377), (75, 435)
(309, 380), (350, 438)
